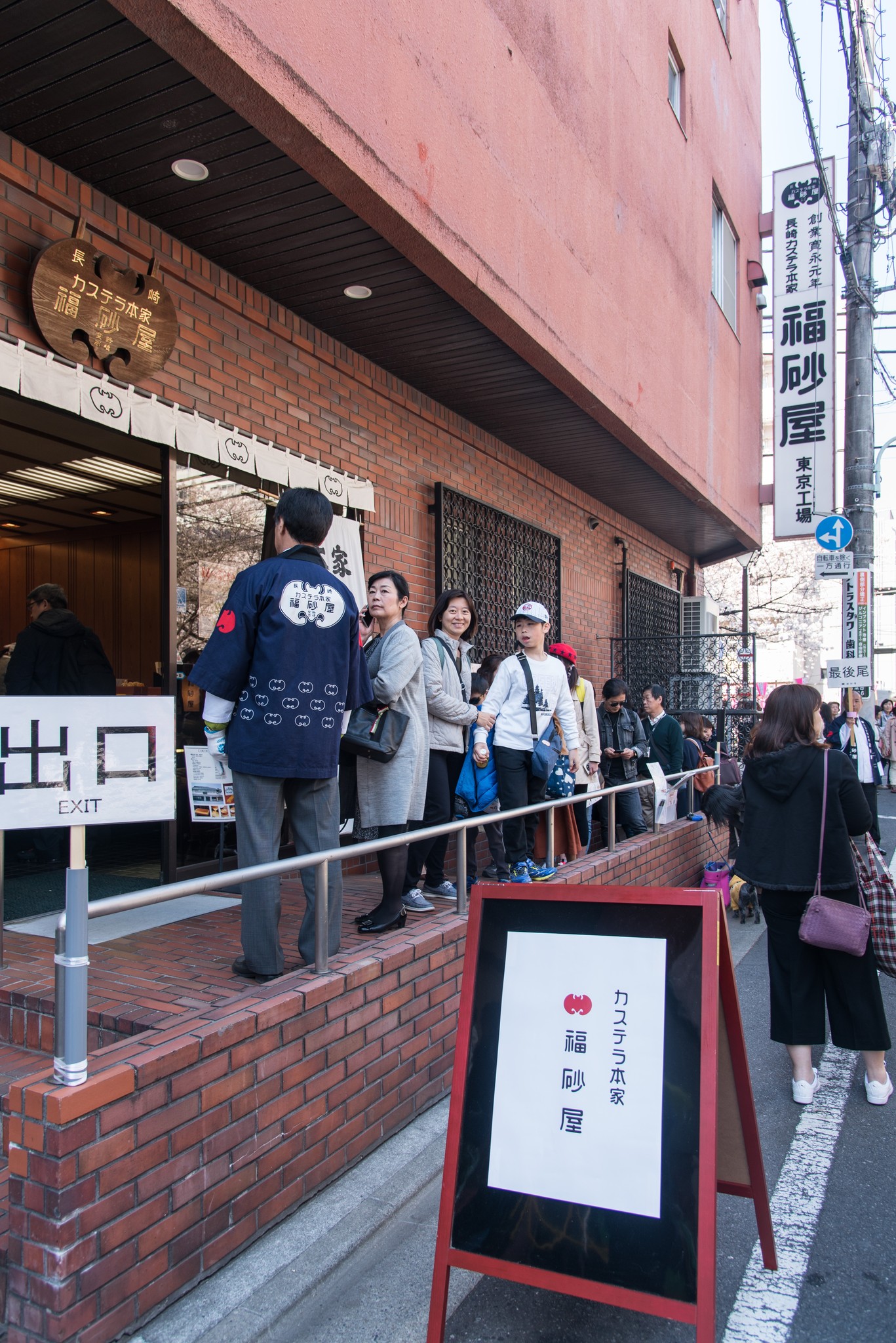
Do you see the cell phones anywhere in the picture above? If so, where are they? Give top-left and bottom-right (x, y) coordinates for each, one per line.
(614, 744), (625, 750)
(361, 604), (374, 628)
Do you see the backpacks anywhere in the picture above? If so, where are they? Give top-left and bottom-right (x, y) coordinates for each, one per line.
(685, 738), (715, 793)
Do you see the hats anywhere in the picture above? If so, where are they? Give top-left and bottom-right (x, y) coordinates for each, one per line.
(549, 643), (577, 665)
(510, 601), (550, 624)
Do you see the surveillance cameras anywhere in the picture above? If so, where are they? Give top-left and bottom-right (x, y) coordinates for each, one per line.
(588, 517), (599, 531)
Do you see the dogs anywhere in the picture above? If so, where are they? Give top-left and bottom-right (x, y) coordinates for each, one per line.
(727, 877), (762, 925)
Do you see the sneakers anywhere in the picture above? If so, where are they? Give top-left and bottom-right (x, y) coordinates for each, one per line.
(401, 888), (435, 911)
(526, 858), (558, 881)
(498, 878), (511, 883)
(509, 862), (533, 884)
(482, 859), (498, 878)
(864, 1060), (893, 1105)
(791, 1067), (821, 1104)
(451, 875), (478, 893)
(421, 880), (468, 900)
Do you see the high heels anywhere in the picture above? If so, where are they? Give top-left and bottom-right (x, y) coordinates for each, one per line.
(357, 903), (407, 933)
(354, 913), (374, 924)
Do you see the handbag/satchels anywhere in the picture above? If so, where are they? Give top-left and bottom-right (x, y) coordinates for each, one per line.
(530, 716), (563, 780)
(798, 894), (872, 956)
(545, 754), (575, 800)
(706, 742), (741, 786)
(849, 832), (896, 980)
(338, 699), (411, 764)
(700, 860), (733, 908)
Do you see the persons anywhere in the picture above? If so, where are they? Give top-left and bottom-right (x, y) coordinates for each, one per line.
(675, 711), (703, 819)
(734, 684), (894, 1105)
(4, 582), (117, 696)
(595, 678), (649, 847)
(877, 699), (894, 727)
(472, 601), (581, 884)
(882, 707), (896, 794)
(547, 643), (602, 865)
(874, 705), (889, 789)
(636, 708), (649, 722)
(828, 702), (840, 720)
(701, 715), (729, 786)
(354, 572), (430, 932)
(187, 486), (378, 983)
(398, 590), (497, 912)
(699, 782), (762, 906)
(819, 701), (833, 738)
(469, 654), (507, 879)
(827, 690), (886, 855)
(635, 684), (684, 828)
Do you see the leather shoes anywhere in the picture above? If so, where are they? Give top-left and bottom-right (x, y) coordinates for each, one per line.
(872, 846), (887, 855)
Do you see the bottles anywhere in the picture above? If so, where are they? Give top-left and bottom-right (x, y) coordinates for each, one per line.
(476, 749), (488, 768)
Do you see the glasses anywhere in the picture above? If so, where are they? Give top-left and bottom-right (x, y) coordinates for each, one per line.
(607, 699), (627, 707)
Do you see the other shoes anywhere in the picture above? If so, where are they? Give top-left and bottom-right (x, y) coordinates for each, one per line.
(876, 784), (889, 789)
(232, 954), (283, 984)
(891, 787), (896, 793)
